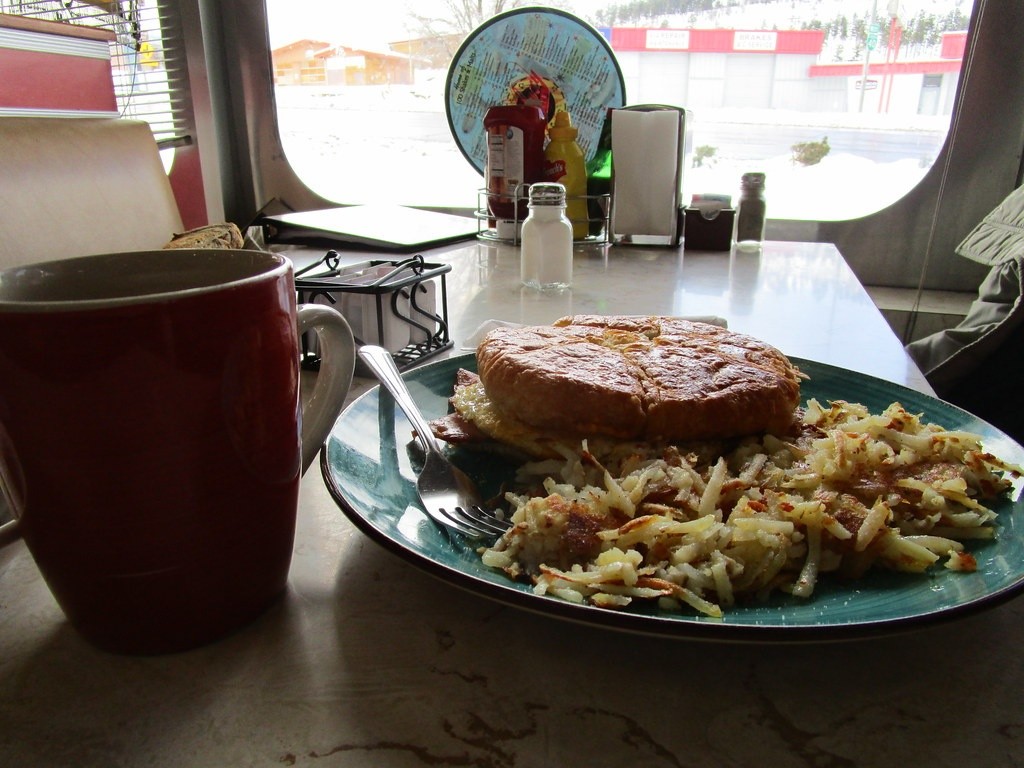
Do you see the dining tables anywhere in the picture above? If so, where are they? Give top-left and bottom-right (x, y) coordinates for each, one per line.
(0, 235), (1023, 768)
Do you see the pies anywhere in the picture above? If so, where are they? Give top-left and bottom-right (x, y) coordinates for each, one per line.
(475, 314), (799, 437)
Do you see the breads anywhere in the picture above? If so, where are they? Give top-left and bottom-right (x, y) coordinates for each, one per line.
(161, 222), (243, 250)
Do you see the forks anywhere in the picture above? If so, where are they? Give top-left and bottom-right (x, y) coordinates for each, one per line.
(358, 345), (515, 537)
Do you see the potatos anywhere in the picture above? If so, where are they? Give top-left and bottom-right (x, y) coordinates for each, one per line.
(479, 399), (1018, 616)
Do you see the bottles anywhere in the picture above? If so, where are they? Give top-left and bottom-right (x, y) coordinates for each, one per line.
(734, 174), (766, 253)
(521, 182), (573, 289)
(544, 111), (590, 240)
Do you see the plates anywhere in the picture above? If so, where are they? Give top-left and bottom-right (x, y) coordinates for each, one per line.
(320, 352), (1024, 639)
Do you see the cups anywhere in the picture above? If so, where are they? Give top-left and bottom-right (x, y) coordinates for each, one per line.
(0, 248), (354, 657)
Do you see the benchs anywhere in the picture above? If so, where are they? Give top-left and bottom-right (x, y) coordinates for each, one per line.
(0, 116), (184, 270)
